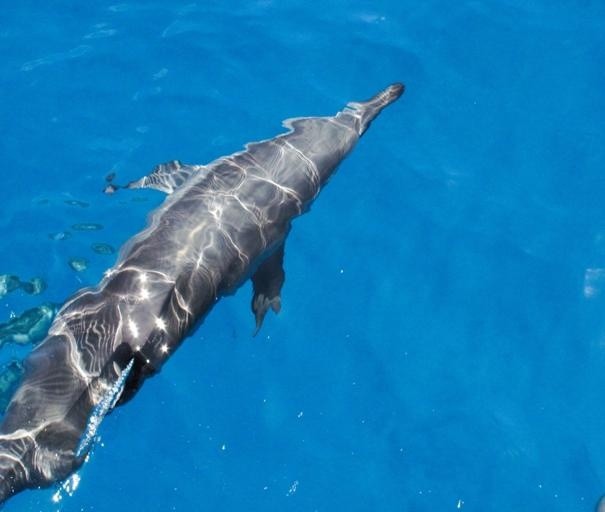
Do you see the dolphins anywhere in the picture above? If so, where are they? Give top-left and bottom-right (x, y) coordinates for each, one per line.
(0, 82), (406, 505)
